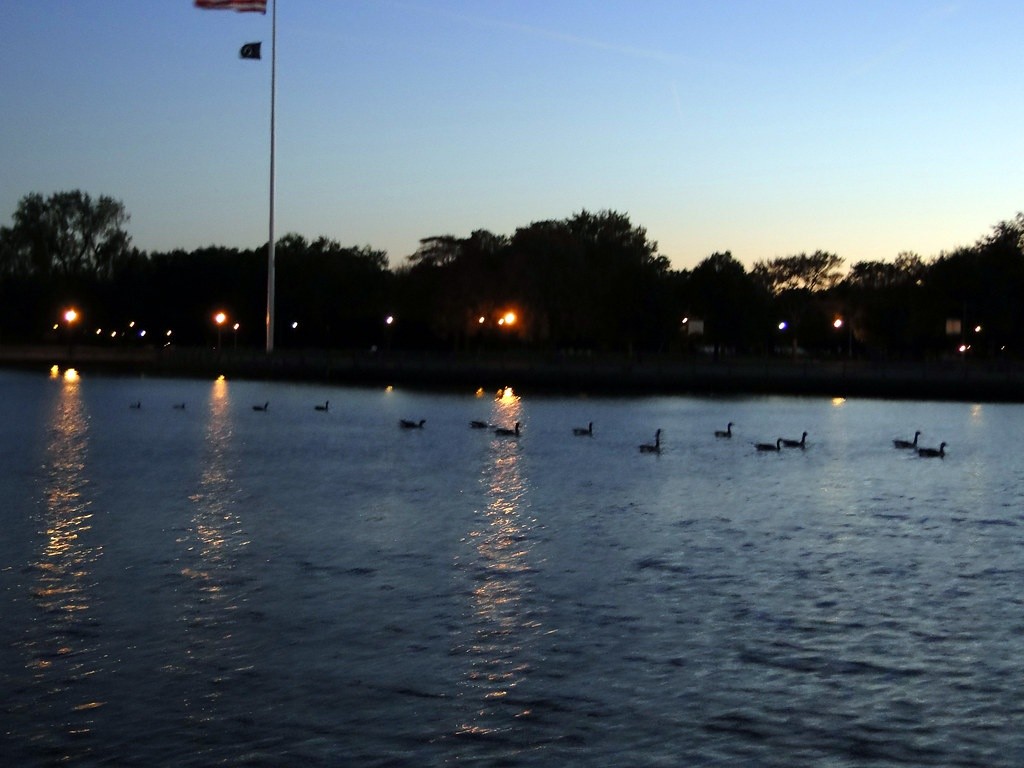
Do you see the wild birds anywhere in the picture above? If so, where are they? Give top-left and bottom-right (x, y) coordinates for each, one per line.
(468, 421), (489, 429)
(493, 421), (520, 439)
(891, 429), (950, 460)
(640, 427), (666, 455)
(753, 431), (809, 455)
(252, 401), (269, 412)
(127, 401), (141, 410)
(571, 420), (594, 438)
(714, 421), (736, 440)
(172, 402), (186, 411)
(314, 400), (330, 414)
(399, 419), (427, 431)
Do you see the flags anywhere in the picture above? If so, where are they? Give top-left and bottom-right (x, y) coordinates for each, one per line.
(193, 0), (267, 14)
(239, 41), (262, 60)
